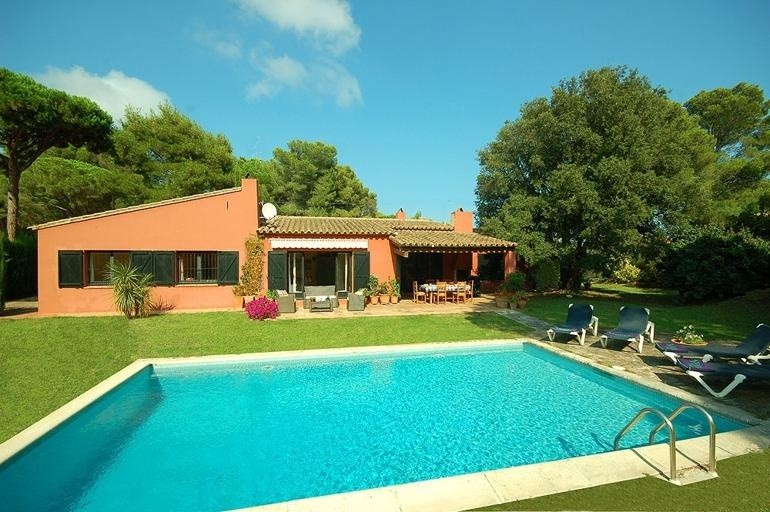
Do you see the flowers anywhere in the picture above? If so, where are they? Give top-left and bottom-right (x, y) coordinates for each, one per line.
(245, 296), (280, 321)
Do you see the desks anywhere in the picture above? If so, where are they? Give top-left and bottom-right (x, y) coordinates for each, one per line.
(310, 297), (333, 312)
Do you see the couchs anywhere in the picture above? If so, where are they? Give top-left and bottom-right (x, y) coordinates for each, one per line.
(303, 285), (339, 309)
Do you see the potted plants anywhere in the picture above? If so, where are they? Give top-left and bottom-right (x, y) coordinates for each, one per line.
(495, 271), (530, 309)
(364, 274), (401, 305)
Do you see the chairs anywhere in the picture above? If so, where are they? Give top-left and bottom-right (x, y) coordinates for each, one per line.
(655, 323), (770, 398)
(412, 278), (474, 305)
(347, 288), (366, 311)
(545, 301), (654, 353)
(273, 288), (296, 313)
